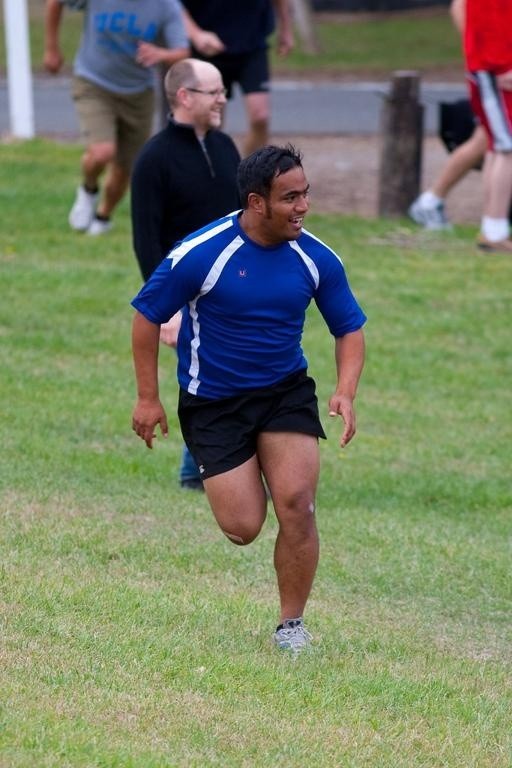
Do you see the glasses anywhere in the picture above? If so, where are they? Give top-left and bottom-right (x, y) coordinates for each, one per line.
(186, 87), (228, 99)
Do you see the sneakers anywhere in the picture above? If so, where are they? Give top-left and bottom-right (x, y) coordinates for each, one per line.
(272, 617), (313, 661)
(87, 219), (113, 235)
(68, 183), (100, 232)
(475, 231), (512, 256)
(407, 193), (453, 234)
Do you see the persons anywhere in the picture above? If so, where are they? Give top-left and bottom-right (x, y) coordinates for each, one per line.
(128, 143), (367, 657)
(128, 56), (244, 491)
(43, 1), (193, 238)
(177, 1), (299, 159)
(404, 0), (512, 253)
